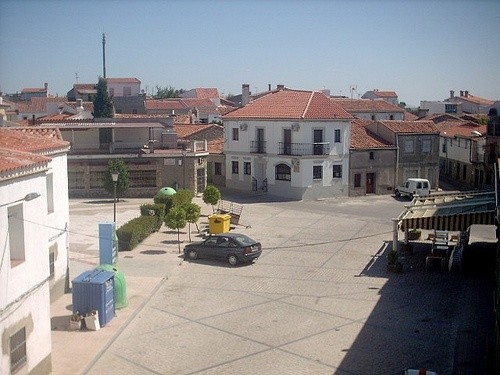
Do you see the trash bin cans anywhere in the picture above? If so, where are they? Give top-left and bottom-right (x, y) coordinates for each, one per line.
(208, 213), (232, 235)
(72, 269), (116, 326)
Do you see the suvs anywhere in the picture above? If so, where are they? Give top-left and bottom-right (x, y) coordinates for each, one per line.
(395, 178), (431, 200)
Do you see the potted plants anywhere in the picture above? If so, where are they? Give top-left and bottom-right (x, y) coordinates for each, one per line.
(400, 243), (414, 255)
(408, 229), (421, 240)
(387, 250), (403, 273)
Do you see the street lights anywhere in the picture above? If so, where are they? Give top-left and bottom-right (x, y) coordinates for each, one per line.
(110, 167), (120, 223)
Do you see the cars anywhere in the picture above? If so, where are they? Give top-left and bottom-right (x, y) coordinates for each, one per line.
(183, 232), (262, 266)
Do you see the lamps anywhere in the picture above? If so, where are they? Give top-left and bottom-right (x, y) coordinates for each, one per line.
(178, 159), (182, 166)
(471, 131), (487, 139)
(0, 192), (41, 207)
